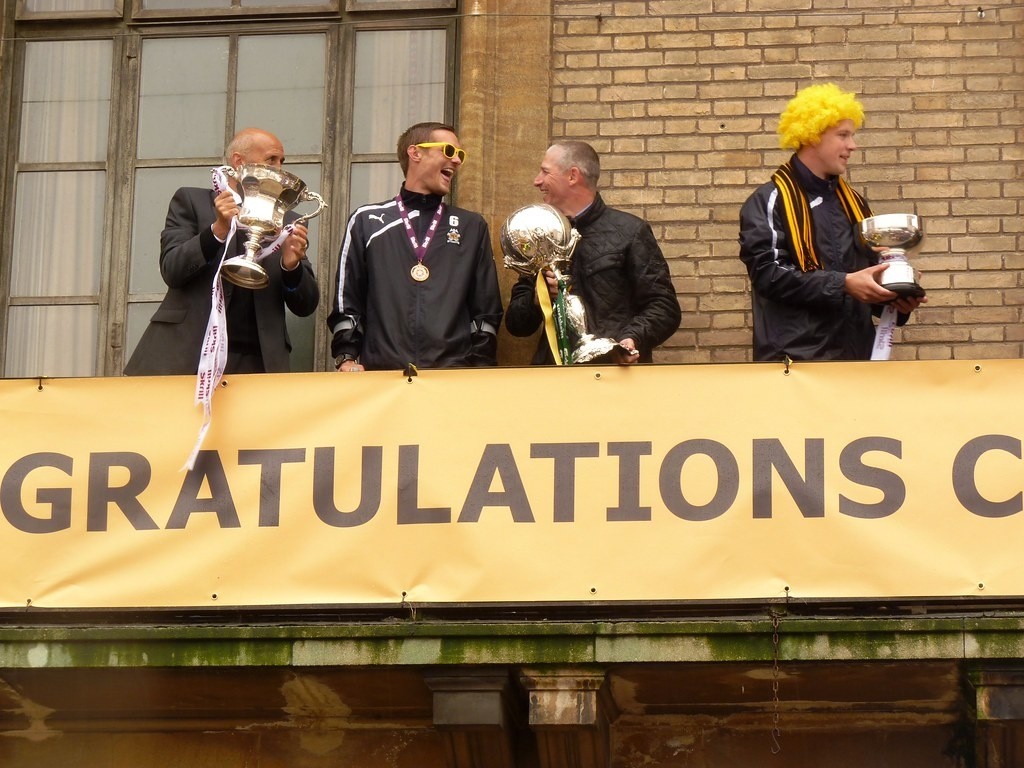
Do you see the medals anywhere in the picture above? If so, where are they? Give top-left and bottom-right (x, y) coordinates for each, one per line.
(410, 261), (429, 282)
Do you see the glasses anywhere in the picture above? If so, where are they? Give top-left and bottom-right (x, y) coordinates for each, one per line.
(415, 142), (466, 165)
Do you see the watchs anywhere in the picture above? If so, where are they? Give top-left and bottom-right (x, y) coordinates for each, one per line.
(334, 353), (356, 370)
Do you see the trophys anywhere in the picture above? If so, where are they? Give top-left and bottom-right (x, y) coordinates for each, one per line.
(209, 162), (328, 290)
(852, 212), (925, 301)
(499, 201), (639, 366)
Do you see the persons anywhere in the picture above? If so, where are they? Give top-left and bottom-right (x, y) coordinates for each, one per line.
(733, 84), (928, 362)
(326, 122), (504, 372)
(122, 127), (320, 375)
(505, 140), (683, 365)
